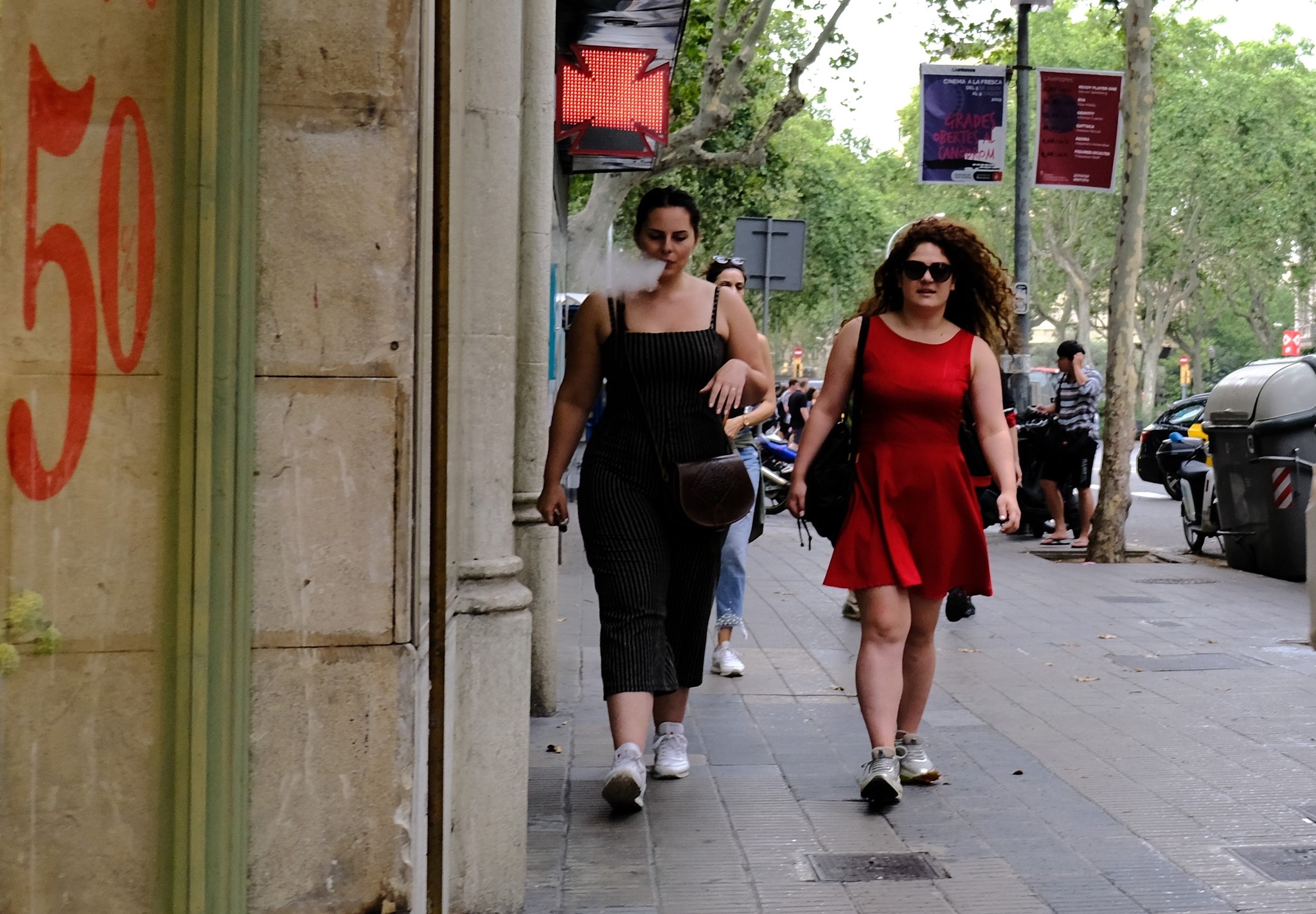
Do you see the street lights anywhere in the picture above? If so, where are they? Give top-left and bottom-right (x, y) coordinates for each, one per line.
(1208, 345), (1216, 391)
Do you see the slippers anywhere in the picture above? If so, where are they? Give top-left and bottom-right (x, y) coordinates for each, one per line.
(1071, 539), (1089, 548)
(1040, 537), (1070, 545)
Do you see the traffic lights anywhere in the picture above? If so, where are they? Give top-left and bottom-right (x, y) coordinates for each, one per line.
(797, 363), (803, 377)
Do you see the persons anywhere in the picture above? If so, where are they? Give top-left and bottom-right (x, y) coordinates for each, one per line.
(535, 192), (768, 817)
(699, 256), (777, 678)
(1032, 340), (1104, 547)
(778, 219), (1023, 806)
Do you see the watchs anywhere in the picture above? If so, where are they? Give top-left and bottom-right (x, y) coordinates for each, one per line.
(743, 414), (752, 426)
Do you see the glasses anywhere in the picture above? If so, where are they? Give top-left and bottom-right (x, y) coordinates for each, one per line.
(710, 255), (746, 266)
(899, 260), (956, 283)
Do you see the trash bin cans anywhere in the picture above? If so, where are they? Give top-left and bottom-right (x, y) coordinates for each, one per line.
(1203, 355), (1316, 583)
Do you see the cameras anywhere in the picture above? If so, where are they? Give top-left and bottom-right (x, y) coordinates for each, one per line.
(553, 509), (567, 532)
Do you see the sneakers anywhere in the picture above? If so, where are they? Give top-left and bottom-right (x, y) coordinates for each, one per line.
(842, 594), (861, 620)
(601, 741), (647, 813)
(710, 641), (744, 678)
(859, 746), (908, 802)
(895, 730), (941, 786)
(650, 721), (690, 779)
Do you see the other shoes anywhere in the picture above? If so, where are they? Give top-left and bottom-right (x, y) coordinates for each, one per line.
(946, 586), (968, 622)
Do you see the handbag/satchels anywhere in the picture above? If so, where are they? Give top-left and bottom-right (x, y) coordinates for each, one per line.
(669, 454), (755, 532)
(1042, 414), (1092, 455)
(791, 314), (870, 550)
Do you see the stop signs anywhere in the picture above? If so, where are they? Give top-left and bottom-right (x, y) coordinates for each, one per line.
(1178, 356), (1189, 365)
(793, 347), (803, 358)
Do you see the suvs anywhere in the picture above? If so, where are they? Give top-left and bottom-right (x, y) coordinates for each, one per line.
(1134, 392), (1213, 501)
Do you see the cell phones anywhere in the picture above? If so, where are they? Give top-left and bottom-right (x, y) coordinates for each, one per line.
(1026, 406), (1039, 412)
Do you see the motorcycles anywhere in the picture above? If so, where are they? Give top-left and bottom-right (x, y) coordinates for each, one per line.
(754, 427), (800, 515)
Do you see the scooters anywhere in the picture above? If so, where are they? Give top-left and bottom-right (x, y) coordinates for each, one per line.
(961, 405), (1084, 540)
(1157, 434), (1257, 556)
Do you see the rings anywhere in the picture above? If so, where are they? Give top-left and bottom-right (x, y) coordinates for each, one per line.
(731, 388), (736, 391)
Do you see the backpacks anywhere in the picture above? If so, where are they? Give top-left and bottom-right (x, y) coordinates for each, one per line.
(774, 401), (786, 422)
